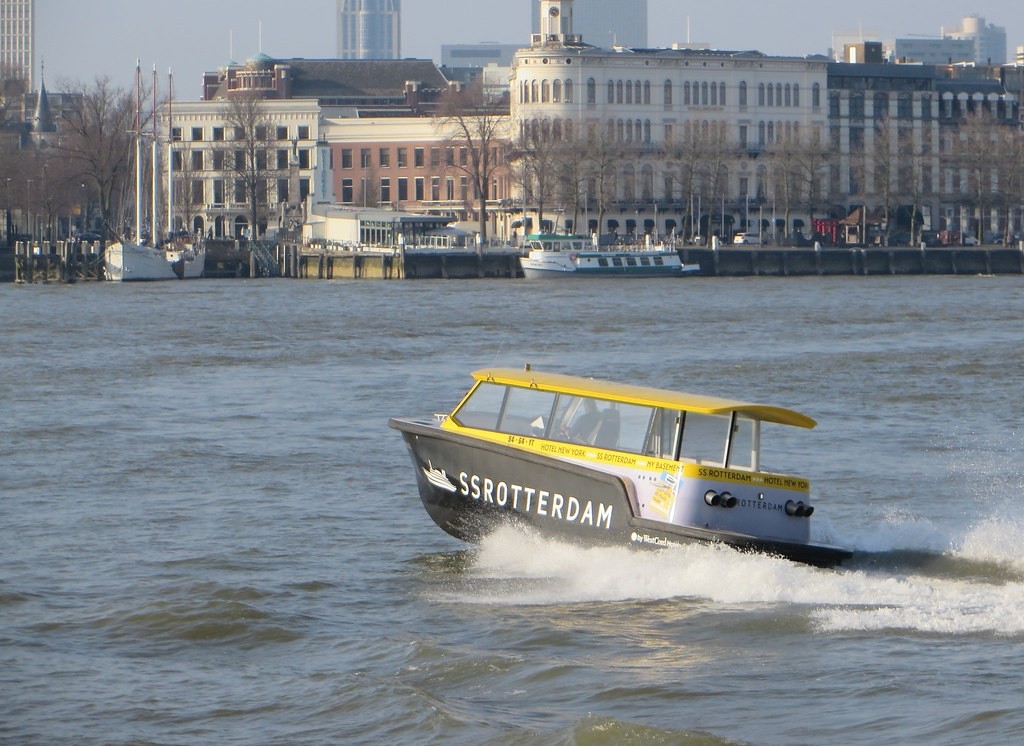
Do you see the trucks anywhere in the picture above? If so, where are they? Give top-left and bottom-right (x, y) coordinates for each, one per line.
(940, 231), (980, 246)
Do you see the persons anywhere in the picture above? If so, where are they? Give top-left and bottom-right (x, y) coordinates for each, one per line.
(643, 229), (647, 238)
(561, 397), (599, 438)
(614, 231), (618, 245)
(962, 233), (966, 246)
(593, 232), (598, 251)
(792, 228), (802, 248)
(240, 226), (245, 240)
(208, 226), (212, 239)
(475, 232), (480, 251)
(1007, 232), (1015, 248)
(513, 228), (517, 240)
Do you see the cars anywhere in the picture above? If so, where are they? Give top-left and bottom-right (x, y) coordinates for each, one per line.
(888, 230), (938, 247)
(734, 233), (766, 245)
(993, 236), (1021, 244)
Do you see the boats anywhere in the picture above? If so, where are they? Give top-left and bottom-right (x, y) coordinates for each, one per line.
(388, 366), (857, 569)
(517, 234), (703, 280)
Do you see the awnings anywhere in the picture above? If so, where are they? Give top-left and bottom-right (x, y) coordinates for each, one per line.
(665, 219), (676, 227)
(897, 206), (924, 225)
(741, 219), (751, 228)
(626, 220), (636, 227)
(776, 219), (784, 226)
(645, 220), (654, 228)
(541, 220), (552, 229)
(759, 220), (768, 226)
(793, 220), (804, 227)
(588, 220), (598, 228)
(565, 220), (573, 228)
(608, 220), (619, 227)
(512, 218), (533, 228)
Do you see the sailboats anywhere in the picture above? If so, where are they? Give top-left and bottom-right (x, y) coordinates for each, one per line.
(103, 56), (204, 282)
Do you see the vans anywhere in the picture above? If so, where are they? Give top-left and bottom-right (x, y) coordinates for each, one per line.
(72, 233), (102, 245)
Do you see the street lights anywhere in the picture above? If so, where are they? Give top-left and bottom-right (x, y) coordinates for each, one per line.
(6, 178), (13, 245)
(27, 180), (34, 234)
